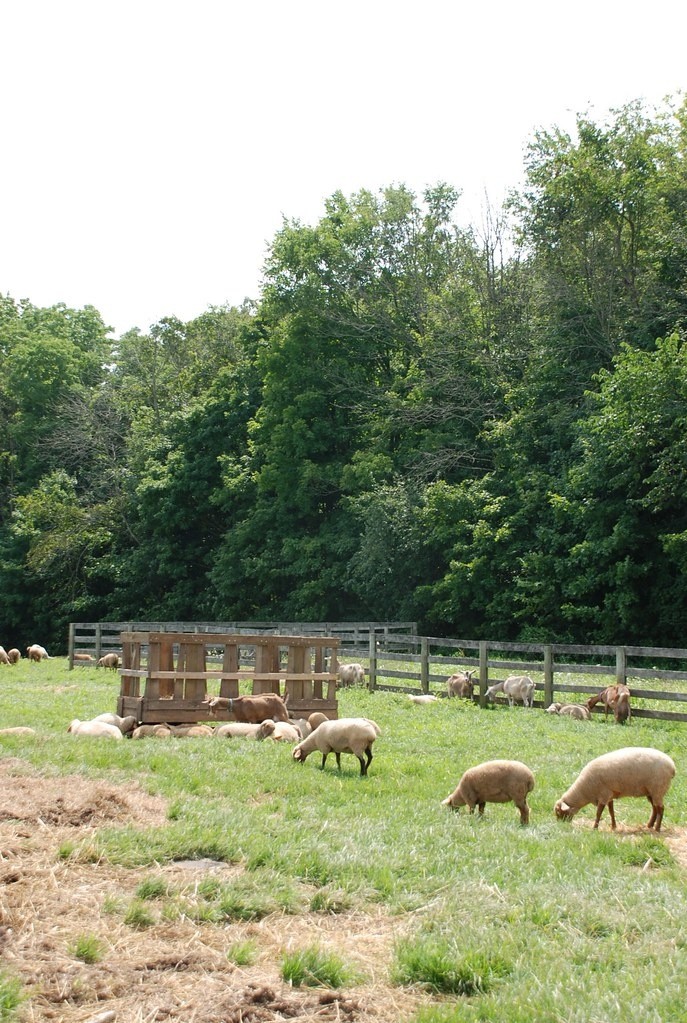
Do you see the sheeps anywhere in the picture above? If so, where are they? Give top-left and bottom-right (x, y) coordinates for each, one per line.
(408, 694), (438, 704)
(445, 670), (477, 702)
(98, 653), (119, 674)
(324, 656), (366, 689)
(293, 718), (381, 777)
(0, 727), (36, 734)
(554, 747), (676, 833)
(587, 683), (632, 725)
(67, 654), (93, 667)
(201, 686), (294, 725)
(26, 644), (53, 663)
(0, 646), (12, 664)
(91, 712), (140, 735)
(441, 759), (535, 825)
(7, 649), (21, 663)
(545, 701), (591, 720)
(483, 676), (537, 707)
(125, 718), (330, 742)
(67, 719), (122, 739)
